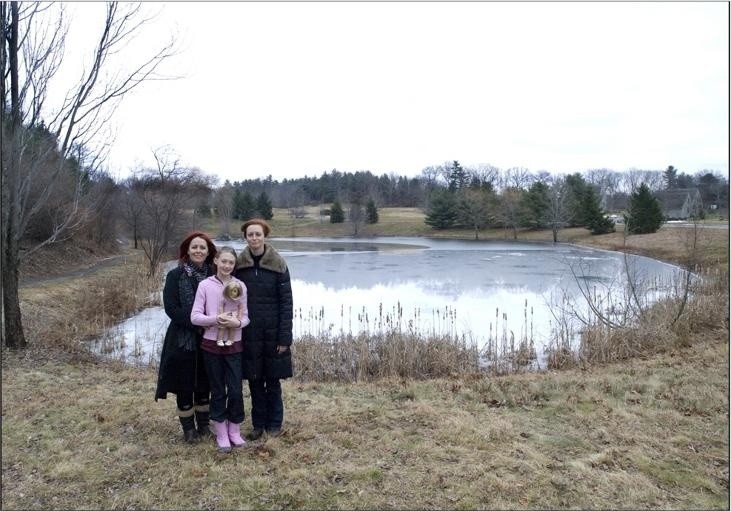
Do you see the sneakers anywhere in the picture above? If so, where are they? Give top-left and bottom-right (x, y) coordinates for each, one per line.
(246, 427), (281, 440)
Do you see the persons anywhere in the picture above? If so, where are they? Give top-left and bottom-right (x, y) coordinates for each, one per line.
(153, 231), (218, 443)
(217, 281), (243, 346)
(190, 246), (249, 453)
(230, 218), (293, 440)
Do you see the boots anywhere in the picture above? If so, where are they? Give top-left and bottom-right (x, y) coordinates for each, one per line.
(177, 404), (210, 445)
(212, 420), (246, 453)
